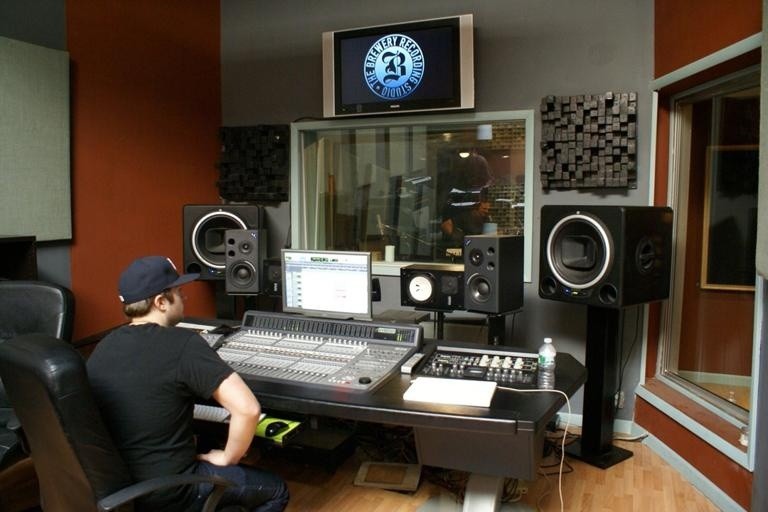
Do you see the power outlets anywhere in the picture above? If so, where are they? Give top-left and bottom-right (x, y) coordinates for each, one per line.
(616, 391), (625, 409)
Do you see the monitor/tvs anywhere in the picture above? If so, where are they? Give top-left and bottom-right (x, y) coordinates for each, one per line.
(280, 248), (372, 322)
(319, 10), (484, 117)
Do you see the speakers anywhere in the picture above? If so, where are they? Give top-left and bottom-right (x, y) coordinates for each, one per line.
(401, 264), (464, 313)
(538, 203), (672, 305)
(464, 233), (525, 317)
(224, 229), (270, 298)
(183, 205), (266, 280)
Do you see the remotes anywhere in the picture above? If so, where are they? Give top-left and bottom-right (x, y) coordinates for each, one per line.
(400, 351), (427, 376)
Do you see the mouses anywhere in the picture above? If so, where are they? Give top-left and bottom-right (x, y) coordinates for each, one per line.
(263, 420), (289, 437)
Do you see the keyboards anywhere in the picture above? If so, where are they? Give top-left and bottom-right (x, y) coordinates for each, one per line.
(193, 403), (265, 431)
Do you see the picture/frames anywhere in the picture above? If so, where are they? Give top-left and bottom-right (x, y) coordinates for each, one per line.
(700, 144), (756, 292)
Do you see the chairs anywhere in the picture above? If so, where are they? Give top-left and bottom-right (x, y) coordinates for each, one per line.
(1, 334), (240, 512)
(0, 279), (76, 512)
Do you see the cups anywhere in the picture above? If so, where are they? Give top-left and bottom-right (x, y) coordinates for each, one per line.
(385, 245), (395, 262)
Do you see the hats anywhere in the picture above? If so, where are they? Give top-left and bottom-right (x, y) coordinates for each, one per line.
(119, 256), (200, 304)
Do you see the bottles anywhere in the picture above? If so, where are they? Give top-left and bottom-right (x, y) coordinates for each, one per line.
(537, 338), (556, 390)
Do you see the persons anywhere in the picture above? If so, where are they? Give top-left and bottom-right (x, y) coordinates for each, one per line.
(451, 198), (495, 241)
(85, 255), (292, 510)
(448, 140), (494, 190)
(441, 182), (481, 236)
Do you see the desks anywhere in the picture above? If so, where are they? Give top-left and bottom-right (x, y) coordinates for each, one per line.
(252, 337), (588, 512)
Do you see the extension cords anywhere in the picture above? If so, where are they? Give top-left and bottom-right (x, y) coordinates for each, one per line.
(500, 479), (530, 495)
